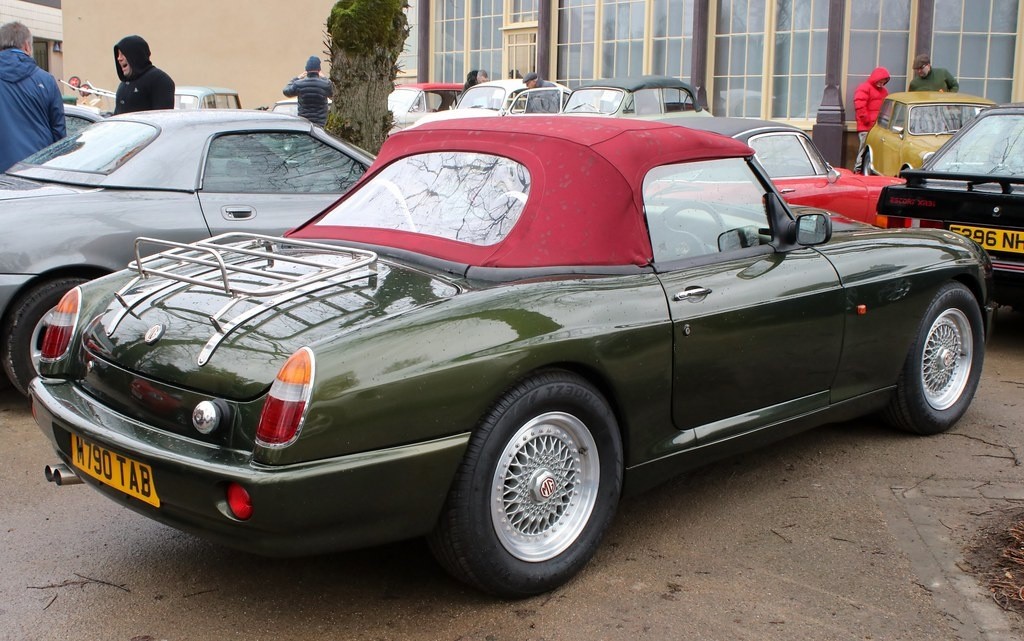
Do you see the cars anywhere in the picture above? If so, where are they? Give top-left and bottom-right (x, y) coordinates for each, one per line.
(175, 86), (240, 110)
(27, 113), (993, 599)
(876, 105), (1023, 322)
(412, 80), (571, 116)
(269, 97), (332, 118)
(861, 91), (999, 179)
(0, 106), (385, 402)
(645, 112), (907, 226)
(389, 82), (467, 128)
(563, 76), (716, 120)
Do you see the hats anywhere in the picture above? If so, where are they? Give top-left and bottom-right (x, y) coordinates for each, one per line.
(913, 54), (930, 69)
(523, 72), (538, 83)
(305, 55), (321, 71)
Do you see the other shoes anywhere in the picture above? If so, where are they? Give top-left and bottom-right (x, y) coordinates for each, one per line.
(854, 170), (863, 174)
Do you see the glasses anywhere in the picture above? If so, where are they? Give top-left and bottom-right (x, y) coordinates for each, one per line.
(914, 64), (928, 72)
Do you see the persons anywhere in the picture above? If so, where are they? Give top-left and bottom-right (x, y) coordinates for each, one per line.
(0, 22), (66, 172)
(113, 35), (174, 114)
(522, 72), (555, 87)
(854, 67), (891, 173)
(283, 56), (333, 127)
(909, 54), (959, 92)
(464, 70), (487, 90)
